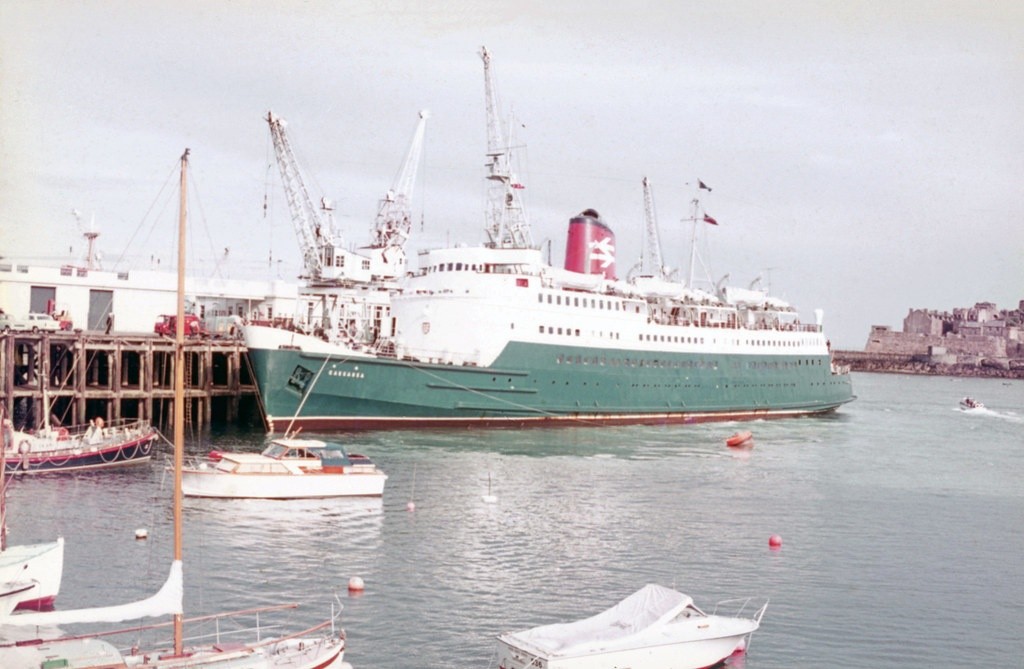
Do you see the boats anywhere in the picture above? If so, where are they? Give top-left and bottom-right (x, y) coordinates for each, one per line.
(242, 48), (857, 433)
(165, 439), (388, 499)
(960, 396), (983, 411)
(489, 583), (770, 669)
(727, 429), (752, 446)
(0, 416), (159, 482)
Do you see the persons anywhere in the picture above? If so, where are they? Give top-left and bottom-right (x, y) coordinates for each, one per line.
(104, 313), (112, 334)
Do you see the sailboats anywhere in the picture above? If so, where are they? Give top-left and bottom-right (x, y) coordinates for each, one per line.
(0, 327), (65, 608)
(0, 147), (353, 669)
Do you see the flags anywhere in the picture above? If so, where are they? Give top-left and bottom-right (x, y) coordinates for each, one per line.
(703, 213), (719, 225)
(699, 180), (712, 192)
